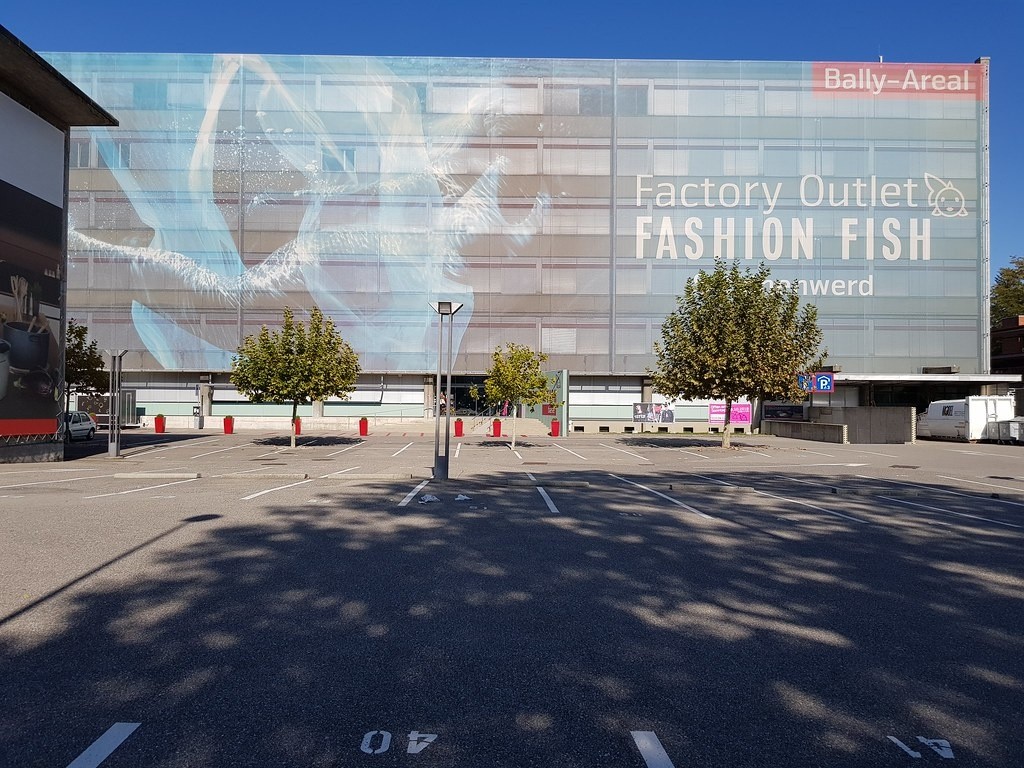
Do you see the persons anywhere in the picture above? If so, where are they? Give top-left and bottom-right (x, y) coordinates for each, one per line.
(633, 403), (673, 422)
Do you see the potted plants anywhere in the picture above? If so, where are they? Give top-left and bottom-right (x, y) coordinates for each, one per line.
(89, 413), (96, 420)
(550, 417), (559, 436)
(455, 418), (462, 437)
(493, 418), (501, 437)
(359, 417), (368, 436)
(292, 416), (301, 434)
(224, 416), (234, 434)
(155, 414), (165, 434)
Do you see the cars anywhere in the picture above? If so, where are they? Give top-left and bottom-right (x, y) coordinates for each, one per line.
(57, 411), (97, 445)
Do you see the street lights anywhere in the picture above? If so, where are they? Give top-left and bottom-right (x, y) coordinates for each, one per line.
(427, 302), (464, 477)
(104, 349), (128, 458)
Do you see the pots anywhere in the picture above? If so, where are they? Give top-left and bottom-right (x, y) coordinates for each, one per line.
(0, 338), (11, 400)
(3, 321), (50, 370)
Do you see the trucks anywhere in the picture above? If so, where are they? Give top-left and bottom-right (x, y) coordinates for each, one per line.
(914, 395), (1015, 441)
